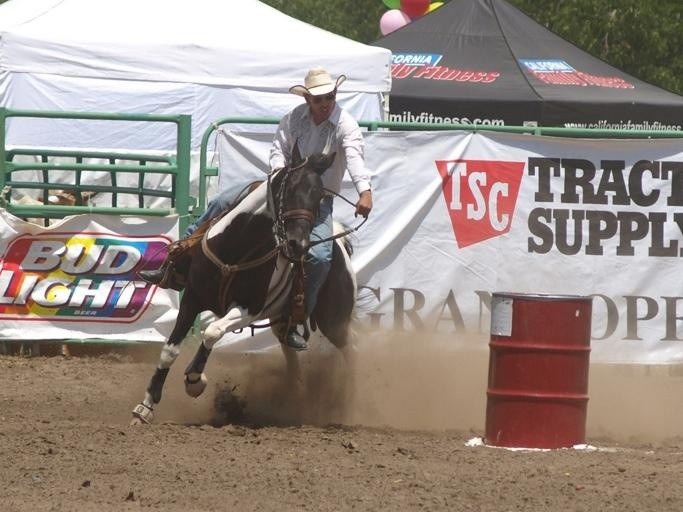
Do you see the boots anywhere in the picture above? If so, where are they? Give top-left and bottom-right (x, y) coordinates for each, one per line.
(279, 313), (309, 351)
(136, 252), (191, 291)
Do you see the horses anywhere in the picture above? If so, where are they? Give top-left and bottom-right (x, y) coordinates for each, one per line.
(131, 137), (357, 427)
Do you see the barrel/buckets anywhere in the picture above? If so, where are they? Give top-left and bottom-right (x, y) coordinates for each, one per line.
(483, 291), (595, 454)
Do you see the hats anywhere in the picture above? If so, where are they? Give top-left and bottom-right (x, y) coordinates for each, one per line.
(289, 70), (346, 96)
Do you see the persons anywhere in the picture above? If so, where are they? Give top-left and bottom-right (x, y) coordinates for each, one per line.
(135, 65), (373, 352)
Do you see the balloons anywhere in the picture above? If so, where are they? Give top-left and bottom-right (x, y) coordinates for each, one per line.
(379, 0), (444, 37)
